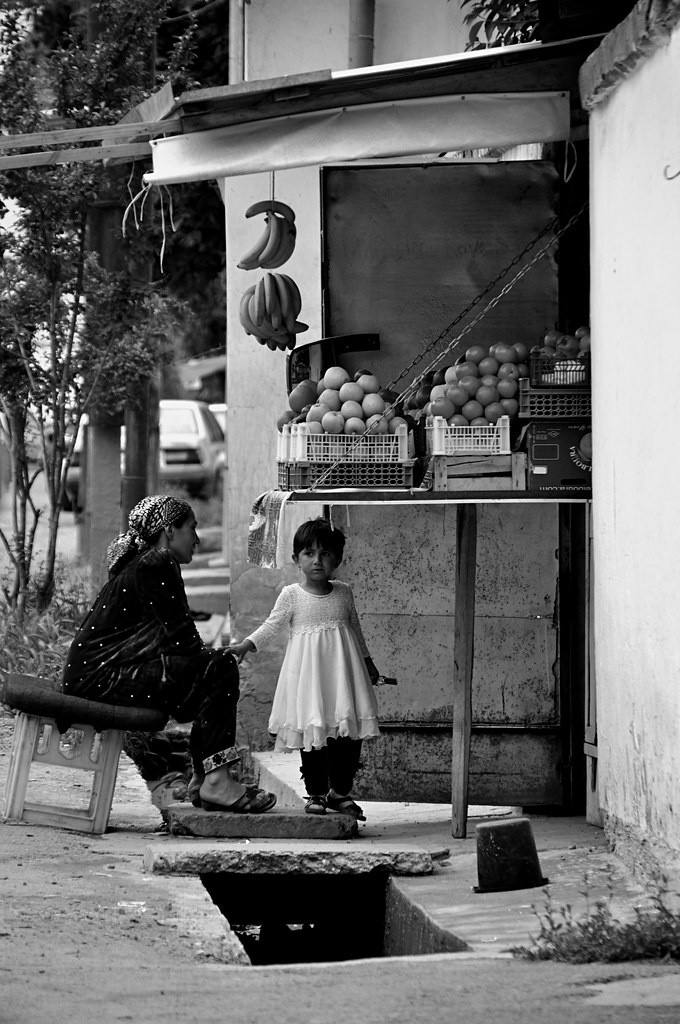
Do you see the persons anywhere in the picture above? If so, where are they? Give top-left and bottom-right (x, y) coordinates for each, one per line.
(61, 494), (276, 814)
(217, 516), (380, 822)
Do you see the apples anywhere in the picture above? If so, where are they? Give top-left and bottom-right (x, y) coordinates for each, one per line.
(529, 326), (590, 359)
(426, 342), (525, 430)
(276, 366), (408, 437)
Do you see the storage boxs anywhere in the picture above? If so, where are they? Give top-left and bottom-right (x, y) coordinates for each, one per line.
(275, 352), (591, 492)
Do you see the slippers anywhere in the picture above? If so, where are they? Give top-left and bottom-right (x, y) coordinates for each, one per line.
(201, 785), (276, 814)
(191, 787), (202, 807)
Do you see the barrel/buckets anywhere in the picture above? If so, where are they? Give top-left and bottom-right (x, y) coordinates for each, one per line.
(473, 818), (549, 893)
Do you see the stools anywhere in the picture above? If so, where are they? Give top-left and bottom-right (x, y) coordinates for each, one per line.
(1, 673), (169, 835)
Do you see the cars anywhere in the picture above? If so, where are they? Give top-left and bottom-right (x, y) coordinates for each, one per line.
(41, 416), (75, 512)
(64, 399), (227, 512)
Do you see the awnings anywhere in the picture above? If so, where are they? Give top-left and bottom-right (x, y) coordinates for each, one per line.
(102, 32), (604, 187)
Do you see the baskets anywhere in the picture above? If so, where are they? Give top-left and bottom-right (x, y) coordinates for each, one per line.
(277, 354), (591, 492)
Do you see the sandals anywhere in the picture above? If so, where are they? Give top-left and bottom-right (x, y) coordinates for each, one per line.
(324, 793), (366, 821)
(303, 796), (328, 815)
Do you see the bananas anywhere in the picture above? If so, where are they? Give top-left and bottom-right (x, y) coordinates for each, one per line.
(237, 201), (297, 270)
(239, 273), (309, 351)
